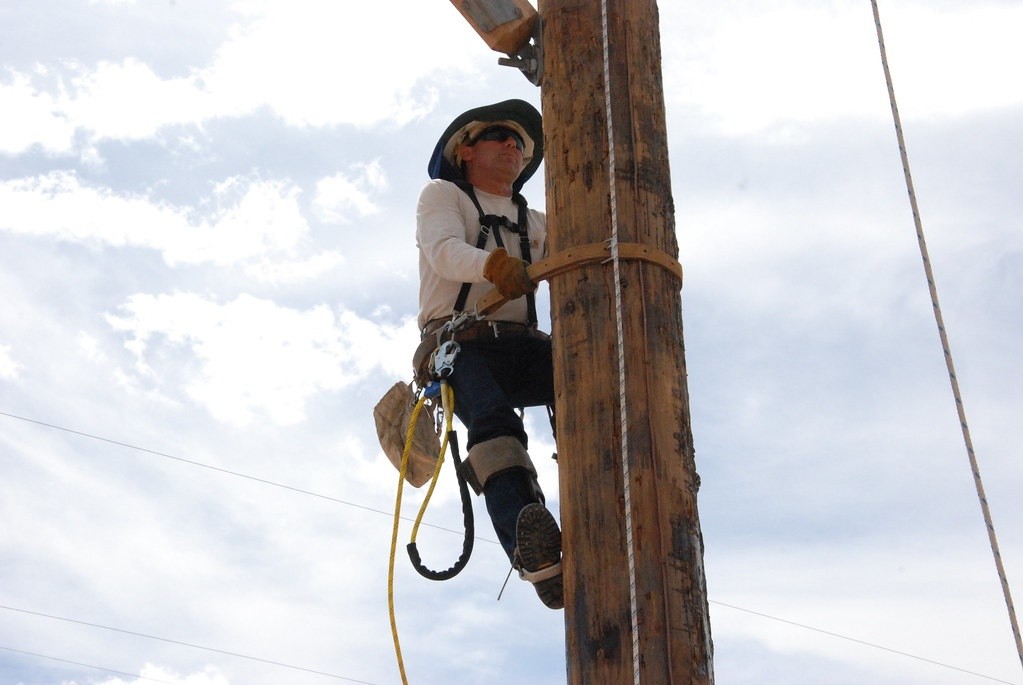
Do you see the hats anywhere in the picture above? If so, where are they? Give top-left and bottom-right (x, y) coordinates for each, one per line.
(428, 99), (543, 193)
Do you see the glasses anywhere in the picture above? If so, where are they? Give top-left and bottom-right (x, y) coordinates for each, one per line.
(466, 125), (524, 156)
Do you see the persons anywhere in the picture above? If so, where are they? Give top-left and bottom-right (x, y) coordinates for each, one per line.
(416, 98), (567, 610)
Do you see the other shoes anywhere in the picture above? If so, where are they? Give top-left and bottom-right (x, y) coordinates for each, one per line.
(516, 503), (563, 609)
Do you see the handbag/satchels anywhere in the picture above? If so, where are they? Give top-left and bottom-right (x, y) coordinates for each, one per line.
(373, 380), (443, 487)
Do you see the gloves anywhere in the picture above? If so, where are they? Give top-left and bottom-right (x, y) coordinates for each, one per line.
(482, 247), (538, 301)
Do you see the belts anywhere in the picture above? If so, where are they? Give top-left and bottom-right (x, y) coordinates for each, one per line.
(450, 320), (551, 342)
